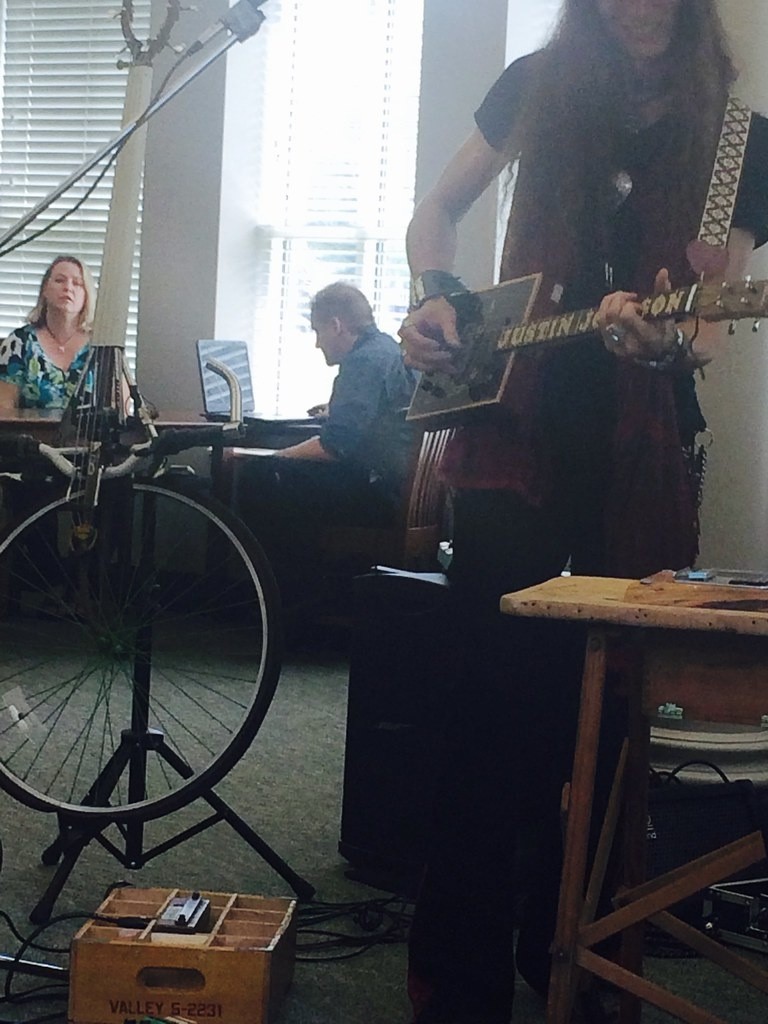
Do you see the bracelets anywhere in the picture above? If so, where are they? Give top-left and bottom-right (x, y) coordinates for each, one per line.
(404, 271), (459, 312)
(634, 326), (689, 372)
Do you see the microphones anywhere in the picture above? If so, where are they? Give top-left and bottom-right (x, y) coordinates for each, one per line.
(185, 1), (267, 55)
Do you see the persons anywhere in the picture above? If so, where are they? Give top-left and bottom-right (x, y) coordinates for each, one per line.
(0, 256), (136, 617)
(266, 282), (422, 630)
(398, 0), (768, 1024)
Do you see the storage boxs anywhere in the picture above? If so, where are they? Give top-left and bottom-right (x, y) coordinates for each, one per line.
(65, 884), (299, 1024)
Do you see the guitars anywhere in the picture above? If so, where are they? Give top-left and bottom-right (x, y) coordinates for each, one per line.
(405, 270), (767, 434)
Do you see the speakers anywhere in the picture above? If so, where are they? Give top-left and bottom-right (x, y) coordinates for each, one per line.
(647, 779), (768, 929)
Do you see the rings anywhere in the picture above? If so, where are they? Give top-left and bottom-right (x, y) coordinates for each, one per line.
(604, 322), (626, 343)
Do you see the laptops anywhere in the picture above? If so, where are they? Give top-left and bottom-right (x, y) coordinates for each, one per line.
(195, 339), (323, 425)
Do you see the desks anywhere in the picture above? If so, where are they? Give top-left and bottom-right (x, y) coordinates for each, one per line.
(499, 571), (768, 1024)
(0, 411), (330, 614)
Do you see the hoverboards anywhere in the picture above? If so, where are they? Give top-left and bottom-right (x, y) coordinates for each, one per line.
(1, 359), (288, 823)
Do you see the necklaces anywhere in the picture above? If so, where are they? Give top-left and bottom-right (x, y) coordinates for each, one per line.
(610, 75), (670, 131)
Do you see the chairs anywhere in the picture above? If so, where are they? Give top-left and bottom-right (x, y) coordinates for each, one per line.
(262, 420), (471, 622)
